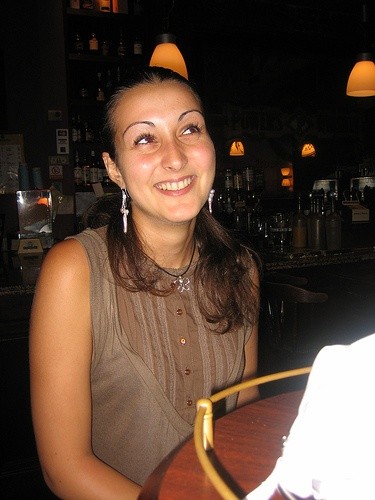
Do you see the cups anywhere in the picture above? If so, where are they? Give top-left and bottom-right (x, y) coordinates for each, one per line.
(271, 216), (289, 244)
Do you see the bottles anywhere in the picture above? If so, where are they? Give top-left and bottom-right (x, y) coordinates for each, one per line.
(71, 29), (265, 236)
(291, 189), (343, 250)
(31, 166), (43, 190)
(18, 162), (29, 190)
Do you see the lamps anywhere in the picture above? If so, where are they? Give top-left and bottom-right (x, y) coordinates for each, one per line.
(147, 0), (190, 80)
(229, 110), (245, 157)
(302, 137), (316, 157)
(346, 0), (375, 98)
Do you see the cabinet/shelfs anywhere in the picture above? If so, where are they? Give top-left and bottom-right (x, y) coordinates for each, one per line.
(0, 0), (145, 194)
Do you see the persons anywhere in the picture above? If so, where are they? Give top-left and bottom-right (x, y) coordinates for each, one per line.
(31, 65), (259, 500)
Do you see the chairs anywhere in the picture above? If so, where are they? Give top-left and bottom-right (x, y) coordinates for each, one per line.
(261, 273), (328, 358)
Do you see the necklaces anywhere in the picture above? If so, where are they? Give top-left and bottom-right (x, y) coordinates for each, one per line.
(148, 240), (197, 292)
